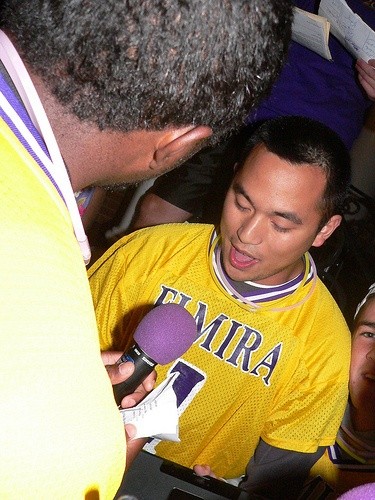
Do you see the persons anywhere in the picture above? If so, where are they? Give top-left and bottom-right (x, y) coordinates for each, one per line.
(279, 285), (375, 500)
(130, 0), (375, 266)
(84, 115), (352, 500)
(0, 0), (288, 500)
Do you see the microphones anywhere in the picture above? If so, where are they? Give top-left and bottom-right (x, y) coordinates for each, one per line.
(111, 304), (197, 409)
